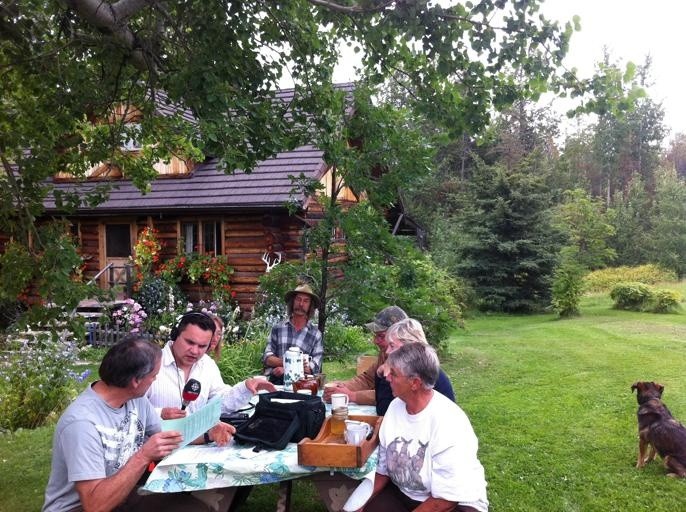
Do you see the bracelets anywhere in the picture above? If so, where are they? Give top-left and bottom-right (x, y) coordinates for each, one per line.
(203, 430), (214, 444)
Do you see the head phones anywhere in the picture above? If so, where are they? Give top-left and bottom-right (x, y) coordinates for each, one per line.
(170, 312), (215, 352)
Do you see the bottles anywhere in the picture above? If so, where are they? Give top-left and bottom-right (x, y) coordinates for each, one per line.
(283, 346), (305, 392)
(331, 406), (349, 435)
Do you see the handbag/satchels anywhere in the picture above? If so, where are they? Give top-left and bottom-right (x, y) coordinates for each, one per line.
(234, 390), (325, 452)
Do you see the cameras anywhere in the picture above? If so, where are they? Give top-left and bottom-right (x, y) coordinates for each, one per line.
(220, 413), (249, 428)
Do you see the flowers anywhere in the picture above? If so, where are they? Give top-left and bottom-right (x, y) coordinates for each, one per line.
(101, 226), (243, 338)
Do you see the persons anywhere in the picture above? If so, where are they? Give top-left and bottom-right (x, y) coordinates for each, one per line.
(261, 283), (324, 386)
(205, 314), (224, 363)
(142, 312), (275, 421)
(322, 305), (408, 407)
(40, 330), (235, 512)
(354, 342), (489, 512)
(374, 317), (455, 416)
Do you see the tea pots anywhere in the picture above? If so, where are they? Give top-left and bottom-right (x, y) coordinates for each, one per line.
(291, 374), (322, 397)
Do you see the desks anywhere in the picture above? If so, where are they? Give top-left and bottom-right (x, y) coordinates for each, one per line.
(156, 384), (379, 512)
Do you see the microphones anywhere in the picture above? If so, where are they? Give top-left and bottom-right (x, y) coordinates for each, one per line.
(182, 378), (201, 413)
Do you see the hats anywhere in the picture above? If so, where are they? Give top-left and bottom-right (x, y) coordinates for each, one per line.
(365, 306), (408, 332)
(284, 284), (321, 308)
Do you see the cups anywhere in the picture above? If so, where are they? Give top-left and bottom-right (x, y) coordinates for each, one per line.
(324, 383), (337, 404)
(331, 392), (350, 409)
(344, 419), (371, 446)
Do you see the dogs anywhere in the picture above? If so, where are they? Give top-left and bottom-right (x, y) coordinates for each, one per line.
(631, 382), (686, 478)
